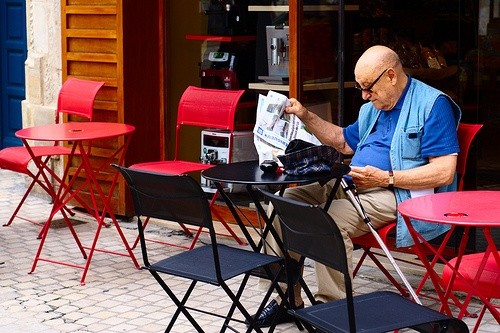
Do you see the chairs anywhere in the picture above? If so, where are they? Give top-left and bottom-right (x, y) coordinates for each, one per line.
(0, 78), (110, 240)
(111, 85), (500, 333)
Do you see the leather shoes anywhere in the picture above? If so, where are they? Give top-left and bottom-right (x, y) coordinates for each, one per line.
(245, 299), (304, 327)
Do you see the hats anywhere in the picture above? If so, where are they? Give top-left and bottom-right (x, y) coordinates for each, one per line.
(277, 144), (339, 175)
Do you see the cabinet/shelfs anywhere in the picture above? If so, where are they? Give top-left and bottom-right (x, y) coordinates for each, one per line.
(248, 0), (359, 212)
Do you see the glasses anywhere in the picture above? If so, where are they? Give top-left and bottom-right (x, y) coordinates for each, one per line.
(355, 69), (387, 94)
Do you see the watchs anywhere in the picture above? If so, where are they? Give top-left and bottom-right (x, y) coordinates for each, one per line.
(388, 170), (395, 191)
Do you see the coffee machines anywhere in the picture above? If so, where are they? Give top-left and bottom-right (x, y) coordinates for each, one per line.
(198, 0), (267, 90)
(258, 22), (290, 85)
(199, 128), (260, 199)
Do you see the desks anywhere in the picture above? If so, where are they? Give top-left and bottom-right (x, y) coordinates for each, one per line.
(396, 191), (500, 333)
(202, 159), (351, 333)
(14, 122), (141, 285)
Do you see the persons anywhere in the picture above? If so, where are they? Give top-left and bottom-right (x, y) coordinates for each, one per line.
(245, 46), (462, 328)
